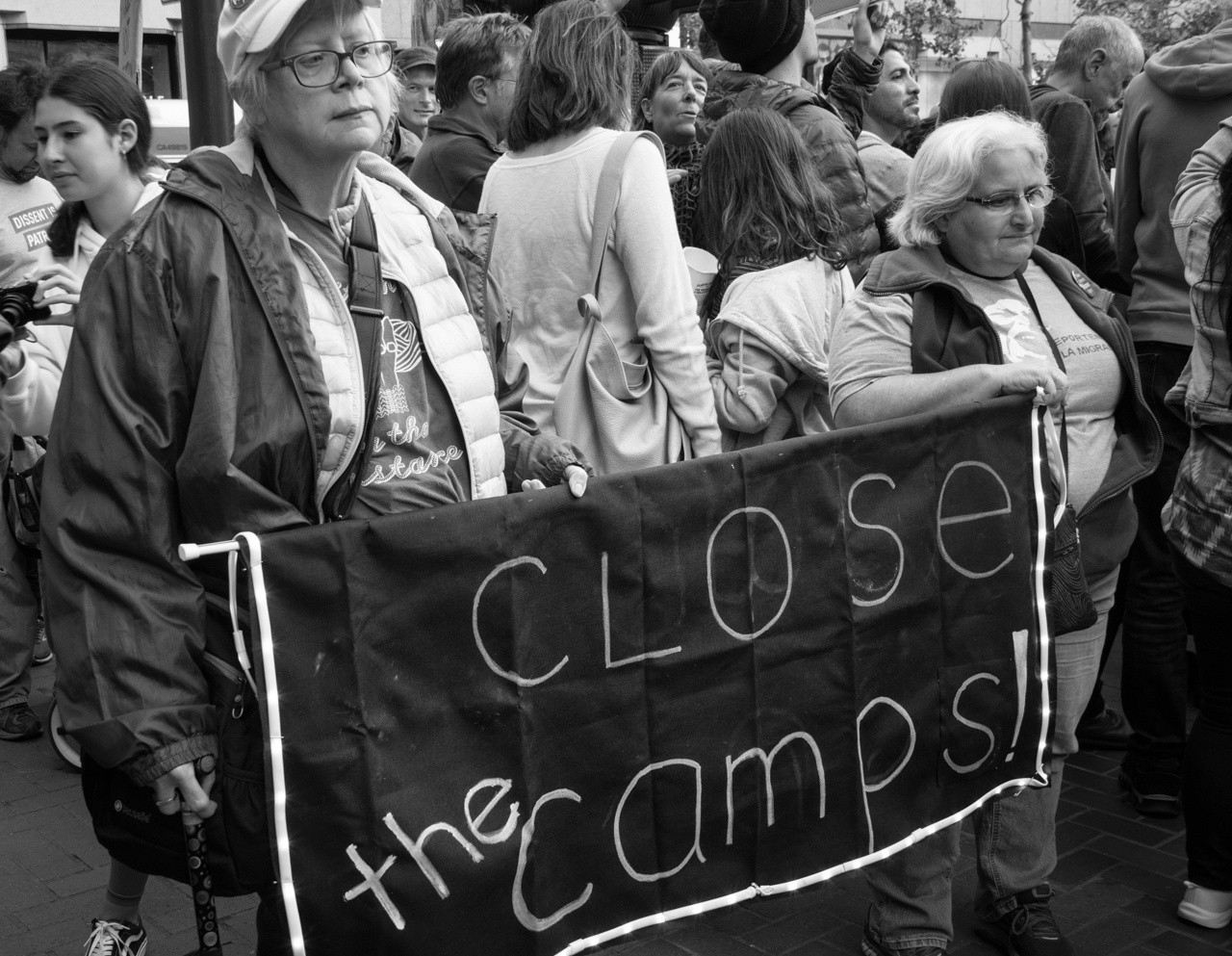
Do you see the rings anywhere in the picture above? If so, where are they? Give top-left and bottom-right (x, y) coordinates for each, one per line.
(156, 791), (178, 806)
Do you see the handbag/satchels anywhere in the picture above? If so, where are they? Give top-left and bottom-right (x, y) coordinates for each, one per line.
(1, 434), (47, 548)
(1048, 501), (1098, 639)
(78, 563), (276, 899)
(552, 293), (694, 478)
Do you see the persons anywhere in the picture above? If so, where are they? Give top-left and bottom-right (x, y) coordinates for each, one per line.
(829, 113), (1162, 956)
(39, 0), (596, 956)
(0, 0), (1232, 956)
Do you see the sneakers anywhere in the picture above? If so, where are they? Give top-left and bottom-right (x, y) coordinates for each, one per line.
(85, 912), (149, 956)
(0, 702), (43, 741)
(974, 903), (1074, 956)
(32, 619), (53, 664)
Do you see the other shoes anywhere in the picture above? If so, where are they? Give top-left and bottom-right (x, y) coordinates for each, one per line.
(1121, 769), (1180, 816)
(1177, 880), (1231, 929)
(862, 897), (949, 956)
(1075, 703), (1136, 752)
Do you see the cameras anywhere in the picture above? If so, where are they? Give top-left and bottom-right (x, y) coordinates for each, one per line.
(0, 280), (51, 329)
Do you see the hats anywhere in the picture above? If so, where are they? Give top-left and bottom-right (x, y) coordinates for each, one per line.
(215, 0), (383, 85)
(697, 1), (806, 79)
(394, 46), (439, 74)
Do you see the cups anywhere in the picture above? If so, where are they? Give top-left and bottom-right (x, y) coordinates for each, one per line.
(682, 245), (720, 317)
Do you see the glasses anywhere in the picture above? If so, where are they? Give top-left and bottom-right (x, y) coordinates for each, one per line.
(260, 39), (398, 89)
(965, 185), (1057, 214)
